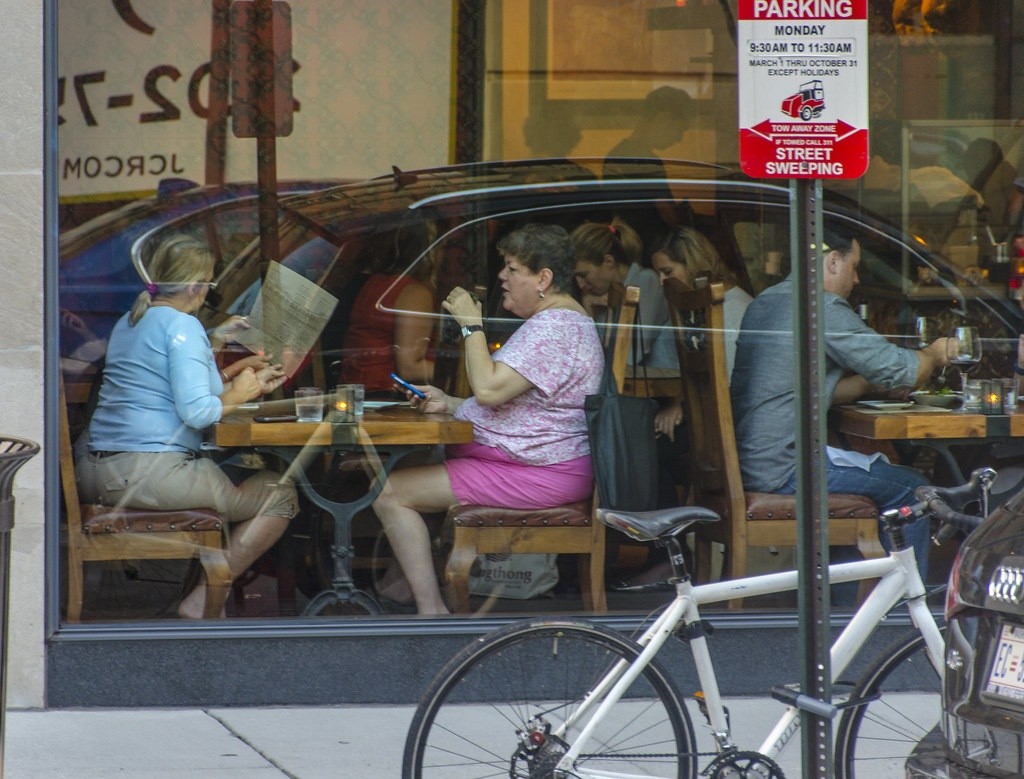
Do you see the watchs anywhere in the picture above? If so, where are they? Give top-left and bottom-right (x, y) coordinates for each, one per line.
(461, 325), (484, 336)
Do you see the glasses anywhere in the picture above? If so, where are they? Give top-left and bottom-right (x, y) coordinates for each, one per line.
(193, 277), (220, 289)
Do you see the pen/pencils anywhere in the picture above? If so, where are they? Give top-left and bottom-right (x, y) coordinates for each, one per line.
(390, 373), (426, 400)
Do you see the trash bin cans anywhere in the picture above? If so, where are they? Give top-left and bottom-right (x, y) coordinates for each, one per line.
(0, 428), (42, 779)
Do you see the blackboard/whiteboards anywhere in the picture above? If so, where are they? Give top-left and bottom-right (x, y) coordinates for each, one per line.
(902, 118), (1024, 297)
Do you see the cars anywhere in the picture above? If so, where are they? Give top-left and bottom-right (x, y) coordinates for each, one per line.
(901, 482), (1023, 779)
(58, 169), (362, 388)
(176, 158), (1024, 461)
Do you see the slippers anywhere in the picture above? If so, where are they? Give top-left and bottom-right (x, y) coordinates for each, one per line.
(367, 586), (420, 613)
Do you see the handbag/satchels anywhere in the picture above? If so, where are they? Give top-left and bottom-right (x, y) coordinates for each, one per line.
(584, 295), (693, 513)
(464, 553), (559, 599)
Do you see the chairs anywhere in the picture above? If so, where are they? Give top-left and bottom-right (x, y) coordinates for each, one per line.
(435, 281), (642, 615)
(57, 359), (235, 623)
(663, 277), (887, 610)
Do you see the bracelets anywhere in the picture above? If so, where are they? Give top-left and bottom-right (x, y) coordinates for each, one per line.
(221, 371), (228, 382)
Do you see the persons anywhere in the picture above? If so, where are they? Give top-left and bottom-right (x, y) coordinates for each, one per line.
(522, 108), (595, 187)
(340, 176), (1024, 616)
(60, 233), (299, 620)
(603, 87), (696, 251)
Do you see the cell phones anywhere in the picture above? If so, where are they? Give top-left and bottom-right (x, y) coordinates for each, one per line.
(389, 374), (426, 400)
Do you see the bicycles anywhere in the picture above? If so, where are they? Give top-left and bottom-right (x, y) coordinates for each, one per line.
(398, 460), (1002, 779)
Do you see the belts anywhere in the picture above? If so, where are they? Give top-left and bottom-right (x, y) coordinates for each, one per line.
(90, 449), (126, 459)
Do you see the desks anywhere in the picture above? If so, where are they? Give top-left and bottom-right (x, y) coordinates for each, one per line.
(825, 391), (1024, 544)
(207, 405), (474, 619)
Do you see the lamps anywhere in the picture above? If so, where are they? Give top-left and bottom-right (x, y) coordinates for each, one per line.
(981, 381), (1004, 415)
(329, 389), (355, 422)
